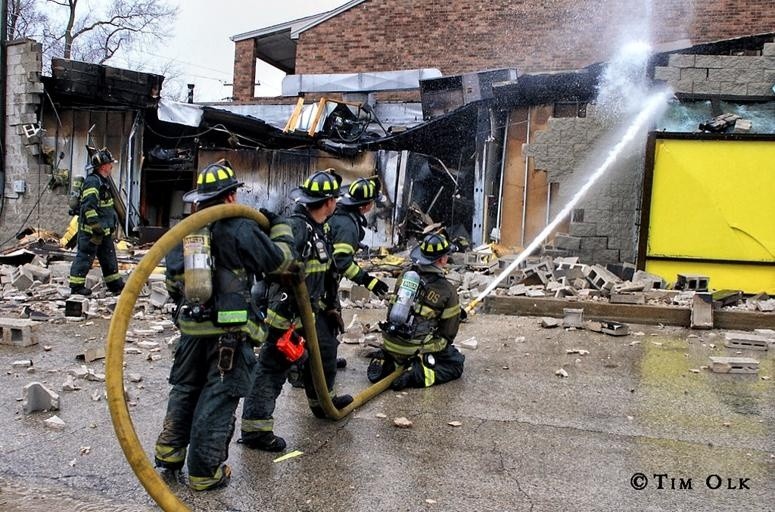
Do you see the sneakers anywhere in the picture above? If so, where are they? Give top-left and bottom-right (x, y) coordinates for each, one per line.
(70, 287), (94, 296)
(367, 350), (386, 383)
(391, 355), (420, 392)
(334, 357), (348, 370)
(111, 283), (127, 297)
(242, 432), (287, 454)
(310, 394), (353, 420)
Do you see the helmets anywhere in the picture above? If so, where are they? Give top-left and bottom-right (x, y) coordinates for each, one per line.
(85, 148), (115, 170)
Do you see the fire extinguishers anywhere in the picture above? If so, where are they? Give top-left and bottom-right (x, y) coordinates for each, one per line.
(180, 226), (213, 319)
(68, 175), (84, 215)
(389, 270), (421, 330)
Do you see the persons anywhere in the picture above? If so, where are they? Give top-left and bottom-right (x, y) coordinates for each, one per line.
(367, 233), (467, 392)
(156, 164), (297, 491)
(320, 178), (388, 371)
(240, 171), (354, 451)
(70, 151), (126, 295)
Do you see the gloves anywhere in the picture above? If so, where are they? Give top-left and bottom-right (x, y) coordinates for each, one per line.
(259, 207), (298, 246)
(327, 310), (345, 337)
(90, 220), (105, 234)
(362, 275), (389, 296)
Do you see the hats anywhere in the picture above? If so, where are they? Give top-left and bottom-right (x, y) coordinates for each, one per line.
(409, 233), (456, 267)
(338, 176), (380, 207)
(182, 162), (245, 204)
(288, 170), (345, 204)
